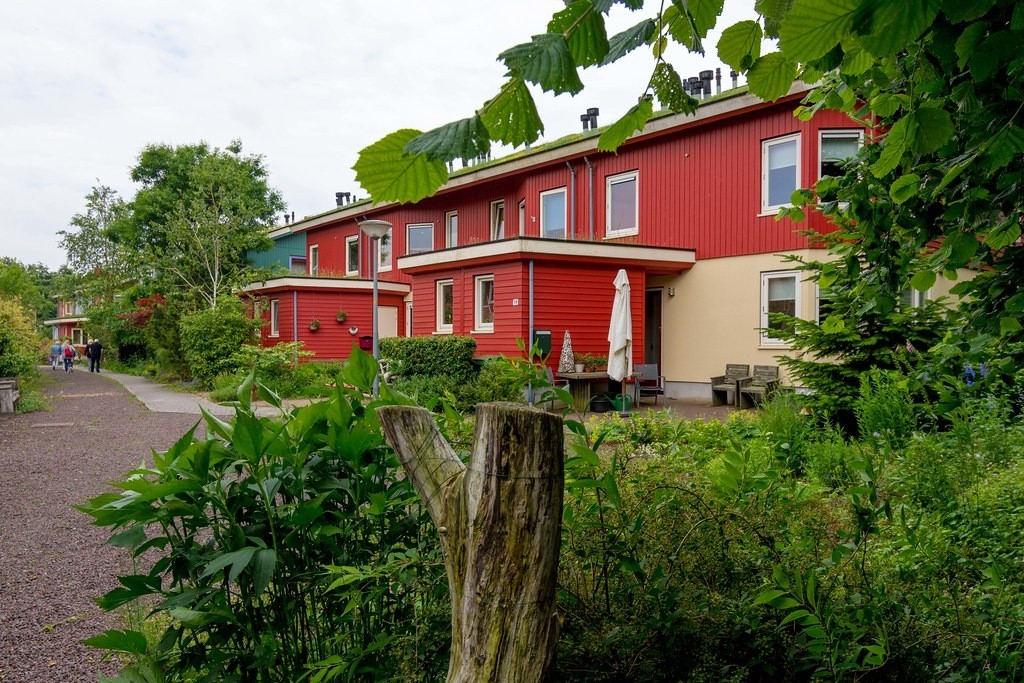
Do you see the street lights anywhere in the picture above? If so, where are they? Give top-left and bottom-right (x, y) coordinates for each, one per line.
(357, 219), (393, 403)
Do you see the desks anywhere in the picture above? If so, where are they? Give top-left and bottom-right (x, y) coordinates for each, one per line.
(556, 372), (637, 414)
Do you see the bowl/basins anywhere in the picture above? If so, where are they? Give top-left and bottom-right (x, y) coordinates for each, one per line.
(591, 400), (608, 412)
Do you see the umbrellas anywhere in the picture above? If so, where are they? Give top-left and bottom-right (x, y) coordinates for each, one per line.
(608, 269), (632, 413)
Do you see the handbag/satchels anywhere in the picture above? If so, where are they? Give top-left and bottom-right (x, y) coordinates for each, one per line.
(65, 348), (74, 357)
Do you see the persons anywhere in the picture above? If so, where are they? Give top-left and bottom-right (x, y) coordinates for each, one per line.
(89, 339), (103, 373)
(84, 339), (94, 371)
(61, 340), (78, 373)
(49, 341), (61, 370)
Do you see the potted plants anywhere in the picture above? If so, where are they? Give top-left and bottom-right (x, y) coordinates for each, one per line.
(337, 311), (347, 322)
(573, 353), (608, 372)
(311, 320), (320, 330)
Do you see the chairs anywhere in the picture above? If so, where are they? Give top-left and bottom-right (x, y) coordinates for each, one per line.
(737, 365), (779, 410)
(710, 364), (749, 407)
(632, 364), (666, 410)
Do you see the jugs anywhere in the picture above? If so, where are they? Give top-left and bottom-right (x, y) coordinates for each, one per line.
(603, 394), (631, 410)
